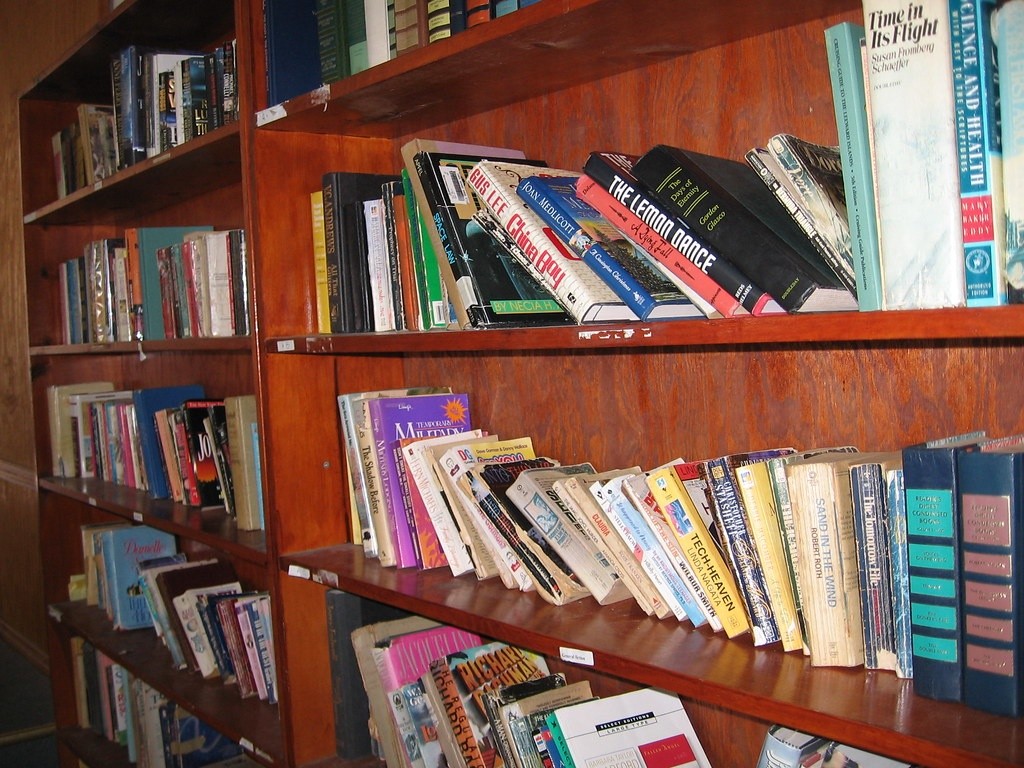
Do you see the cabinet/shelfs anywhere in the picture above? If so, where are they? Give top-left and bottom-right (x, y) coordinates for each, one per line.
(247, 0), (1024, 768)
(0, 0), (290, 768)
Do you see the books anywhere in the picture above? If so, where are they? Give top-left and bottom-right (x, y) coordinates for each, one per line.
(259, 0), (1024, 359)
(57, 220), (253, 344)
(335, 387), (1024, 768)
(42, 32), (240, 205)
(44, 368), (285, 768)
